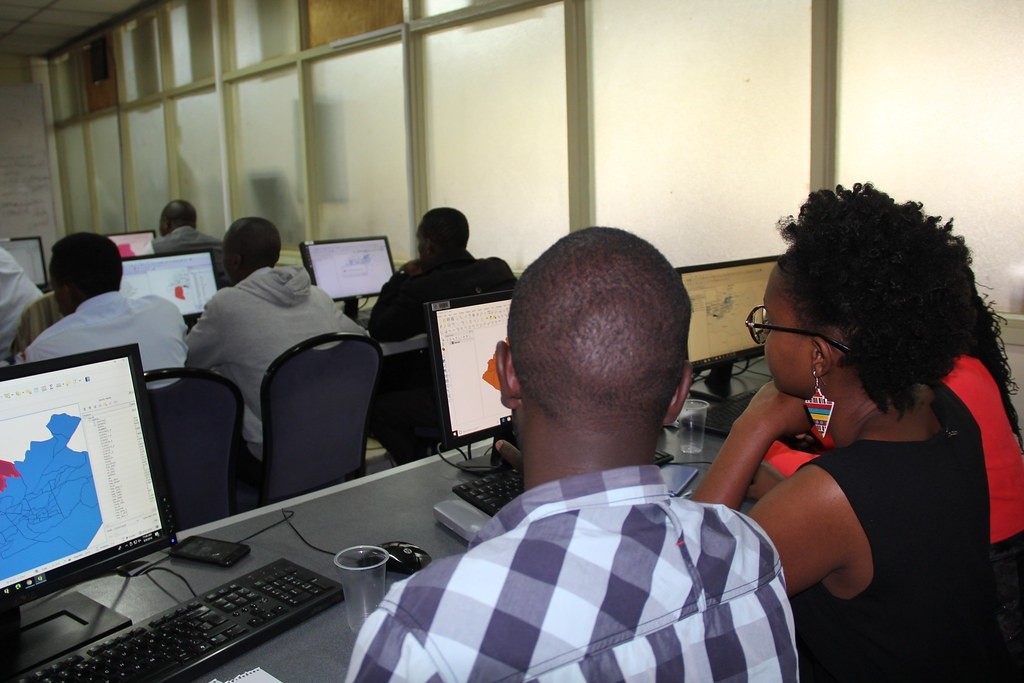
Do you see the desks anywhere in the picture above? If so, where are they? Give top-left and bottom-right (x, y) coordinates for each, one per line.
(0, 308), (1024, 683)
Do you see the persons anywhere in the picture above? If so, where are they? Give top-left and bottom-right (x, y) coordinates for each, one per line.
(0, 245), (44, 367)
(139, 200), (234, 291)
(185, 217), (371, 516)
(344, 227), (799, 683)
(14, 232), (188, 390)
(689, 182), (1024, 683)
(367, 208), (518, 465)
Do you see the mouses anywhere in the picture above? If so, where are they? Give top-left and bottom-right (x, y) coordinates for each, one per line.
(357, 541), (433, 575)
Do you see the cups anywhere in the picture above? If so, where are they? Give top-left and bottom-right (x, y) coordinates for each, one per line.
(678, 399), (709, 454)
(334, 544), (390, 632)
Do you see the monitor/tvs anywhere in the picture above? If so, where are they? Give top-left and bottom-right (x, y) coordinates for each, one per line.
(0, 343), (179, 683)
(675, 254), (784, 402)
(300, 236), (397, 325)
(423, 288), (525, 475)
(0, 230), (218, 337)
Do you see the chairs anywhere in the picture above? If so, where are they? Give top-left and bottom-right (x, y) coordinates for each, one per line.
(144, 368), (245, 538)
(260, 334), (382, 508)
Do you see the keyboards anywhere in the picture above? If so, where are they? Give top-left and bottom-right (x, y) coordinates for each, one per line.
(17, 557), (346, 683)
(681, 386), (762, 438)
(452, 446), (674, 518)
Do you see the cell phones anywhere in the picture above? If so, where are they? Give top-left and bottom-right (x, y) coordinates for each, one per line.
(659, 465), (699, 496)
(168, 535), (251, 567)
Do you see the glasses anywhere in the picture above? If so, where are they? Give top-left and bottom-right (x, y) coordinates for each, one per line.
(745, 304), (851, 355)
